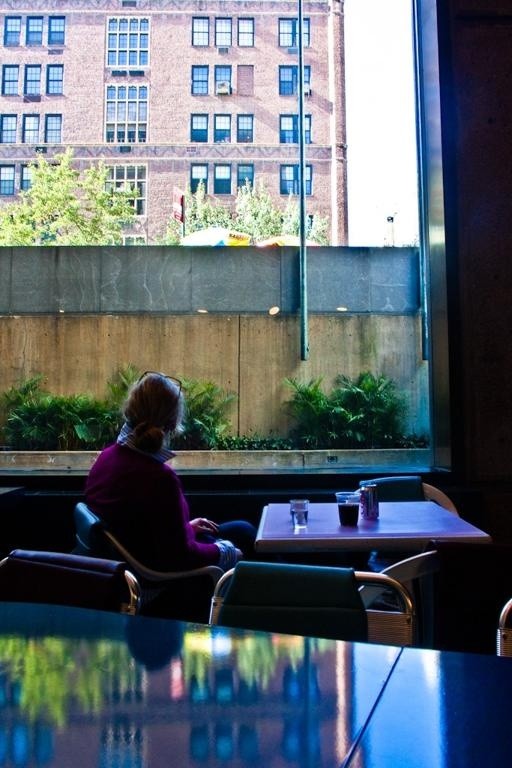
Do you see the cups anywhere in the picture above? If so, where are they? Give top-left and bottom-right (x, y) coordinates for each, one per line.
(336, 492), (362, 526)
(290, 499), (309, 529)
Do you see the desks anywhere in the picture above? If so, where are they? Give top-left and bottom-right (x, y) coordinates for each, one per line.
(254, 501), (492, 648)
(0, 602), (512, 768)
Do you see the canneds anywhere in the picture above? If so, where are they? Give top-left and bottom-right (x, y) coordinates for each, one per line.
(361, 484), (379, 520)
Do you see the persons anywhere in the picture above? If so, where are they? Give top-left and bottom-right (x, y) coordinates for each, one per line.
(84, 371), (258, 625)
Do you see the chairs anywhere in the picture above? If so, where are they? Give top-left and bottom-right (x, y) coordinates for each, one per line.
(496, 598), (512, 657)
(348, 475), (459, 601)
(1, 548), (142, 615)
(208, 561), (416, 648)
(74, 501), (225, 585)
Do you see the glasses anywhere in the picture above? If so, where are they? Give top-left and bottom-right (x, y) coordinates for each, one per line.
(137, 371), (181, 397)
